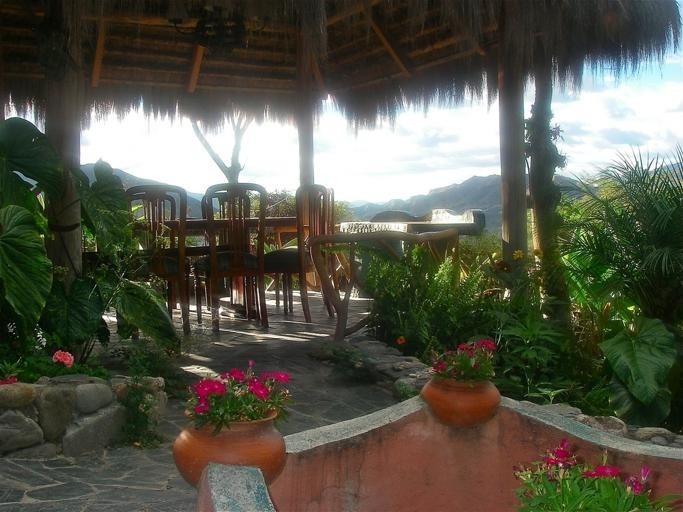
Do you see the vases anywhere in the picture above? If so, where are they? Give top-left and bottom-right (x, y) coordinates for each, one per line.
(169, 408), (289, 491)
(420, 374), (503, 427)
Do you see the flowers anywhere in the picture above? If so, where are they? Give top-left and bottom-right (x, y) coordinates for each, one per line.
(180, 359), (292, 426)
(429, 336), (498, 380)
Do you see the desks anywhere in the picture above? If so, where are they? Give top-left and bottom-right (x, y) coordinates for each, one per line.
(331, 205), (485, 306)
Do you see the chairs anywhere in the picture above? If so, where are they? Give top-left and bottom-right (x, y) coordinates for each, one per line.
(116, 179), (337, 337)
